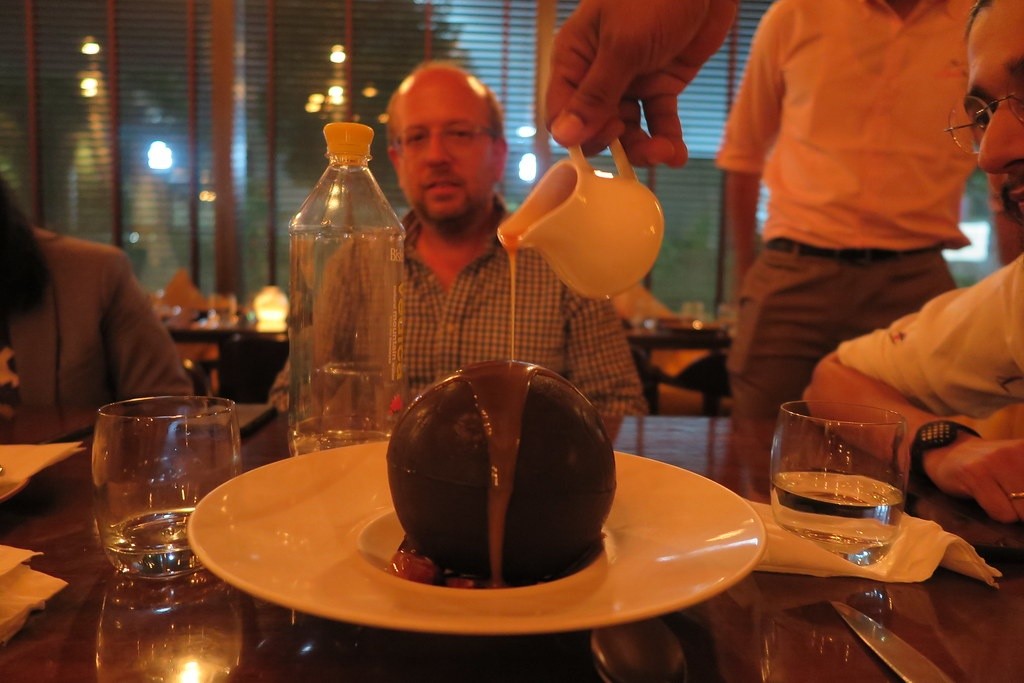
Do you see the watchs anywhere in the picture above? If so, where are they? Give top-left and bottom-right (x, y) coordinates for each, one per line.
(910, 421), (983, 490)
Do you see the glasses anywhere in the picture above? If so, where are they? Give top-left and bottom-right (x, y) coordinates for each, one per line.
(943, 88), (1024, 156)
(385, 119), (499, 161)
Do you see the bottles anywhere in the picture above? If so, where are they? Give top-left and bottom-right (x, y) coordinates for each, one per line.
(287, 122), (394, 458)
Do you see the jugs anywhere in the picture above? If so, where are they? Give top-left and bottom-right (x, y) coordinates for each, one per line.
(497, 138), (665, 304)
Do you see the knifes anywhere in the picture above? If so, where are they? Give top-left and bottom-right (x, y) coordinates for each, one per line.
(832, 600), (953, 683)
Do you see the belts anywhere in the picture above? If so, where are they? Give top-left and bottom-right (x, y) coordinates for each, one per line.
(767, 234), (934, 265)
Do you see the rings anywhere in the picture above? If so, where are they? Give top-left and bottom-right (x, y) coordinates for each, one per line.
(1007, 492), (1024, 499)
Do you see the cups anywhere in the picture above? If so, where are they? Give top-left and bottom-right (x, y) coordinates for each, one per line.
(681, 302), (706, 323)
(770, 401), (908, 565)
(321, 361), (383, 450)
(93, 394), (243, 580)
(207, 292), (238, 328)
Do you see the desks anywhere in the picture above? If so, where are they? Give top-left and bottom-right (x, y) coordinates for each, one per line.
(167, 327), (287, 401)
(0, 405), (1024, 683)
(627, 334), (734, 417)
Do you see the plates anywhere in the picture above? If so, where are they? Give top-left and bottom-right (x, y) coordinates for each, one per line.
(195, 445), (766, 633)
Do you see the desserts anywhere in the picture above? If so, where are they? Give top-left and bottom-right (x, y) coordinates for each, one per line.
(385, 359), (617, 589)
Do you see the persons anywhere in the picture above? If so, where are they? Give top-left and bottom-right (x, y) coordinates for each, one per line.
(0, 167), (190, 442)
(267, 66), (648, 418)
(801, 0), (1024, 522)
(706, 0), (979, 417)
(542, 0), (739, 167)
